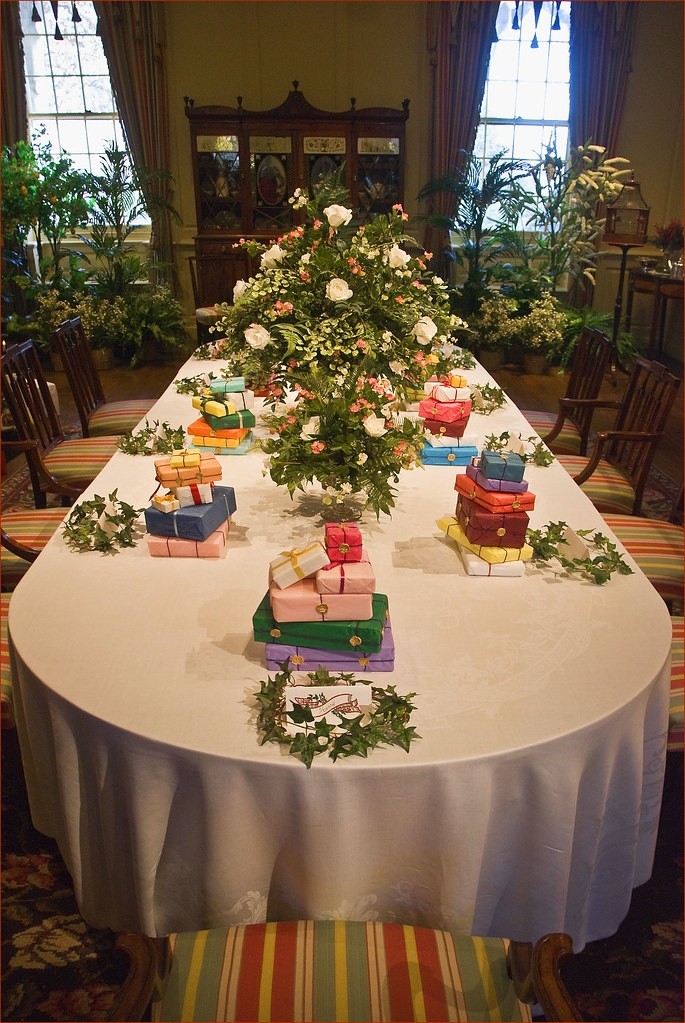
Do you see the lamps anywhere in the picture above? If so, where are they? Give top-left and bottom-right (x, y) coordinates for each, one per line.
(602, 174), (652, 341)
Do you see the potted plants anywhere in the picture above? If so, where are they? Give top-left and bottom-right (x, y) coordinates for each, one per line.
(0, 123), (186, 360)
(410, 131), (633, 304)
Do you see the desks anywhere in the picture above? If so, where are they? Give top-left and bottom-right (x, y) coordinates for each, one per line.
(623, 267), (685, 369)
(9, 339), (672, 954)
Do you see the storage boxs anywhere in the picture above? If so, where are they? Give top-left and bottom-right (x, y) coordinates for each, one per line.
(144, 374), (537, 670)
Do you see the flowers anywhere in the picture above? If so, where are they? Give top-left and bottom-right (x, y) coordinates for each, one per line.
(11, 158), (635, 520)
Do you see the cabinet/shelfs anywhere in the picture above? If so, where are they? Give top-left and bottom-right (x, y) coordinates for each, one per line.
(183, 80), (410, 341)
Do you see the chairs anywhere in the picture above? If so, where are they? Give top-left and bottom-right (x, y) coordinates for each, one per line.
(1, 312), (159, 726)
(104, 920), (583, 1023)
(183, 253), (252, 346)
(520, 328), (685, 749)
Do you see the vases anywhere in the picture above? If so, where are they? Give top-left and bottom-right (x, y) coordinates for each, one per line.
(320, 485), (362, 522)
(90, 349), (113, 370)
(48, 352), (64, 373)
(523, 354), (548, 375)
(478, 348), (505, 370)
(112, 345), (135, 370)
